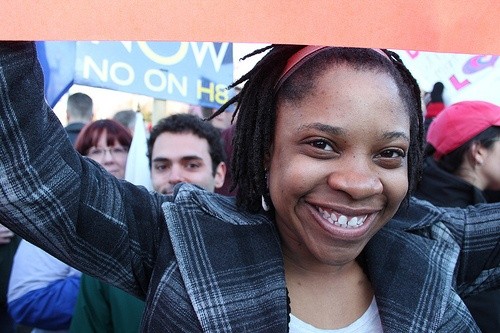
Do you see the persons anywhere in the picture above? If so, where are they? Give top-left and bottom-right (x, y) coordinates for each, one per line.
(0, 40), (499, 333)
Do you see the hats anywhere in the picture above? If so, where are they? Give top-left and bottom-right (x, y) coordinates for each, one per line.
(427, 101), (500, 163)
